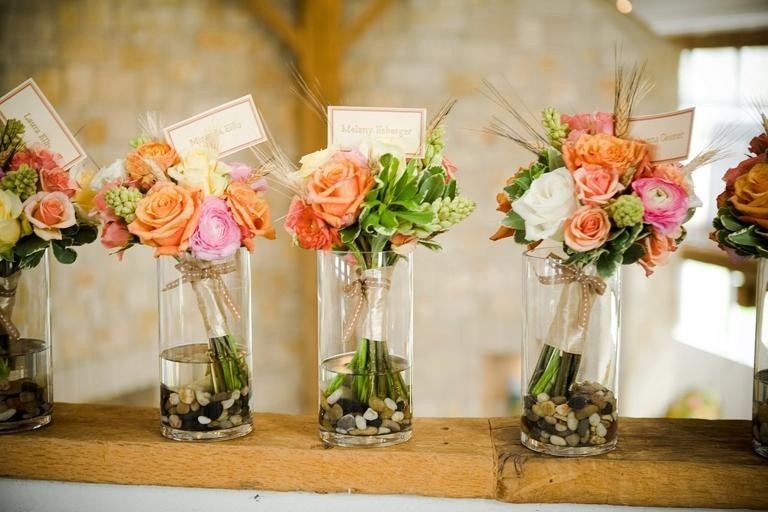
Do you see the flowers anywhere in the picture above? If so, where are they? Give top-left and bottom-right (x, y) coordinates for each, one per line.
(0, 118), (103, 371)
(85, 107), (277, 392)
(461, 37), (745, 395)
(710, 99), (767, 258)
(273, 55), (475, 403)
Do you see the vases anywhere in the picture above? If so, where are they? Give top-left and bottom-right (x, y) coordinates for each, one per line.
(315, 246), (413, 446)
(153, 247), (256, 443)
(751, 255), (768, 464)
(0, 244), (54, 436)
(517, 249), (621, 454)
